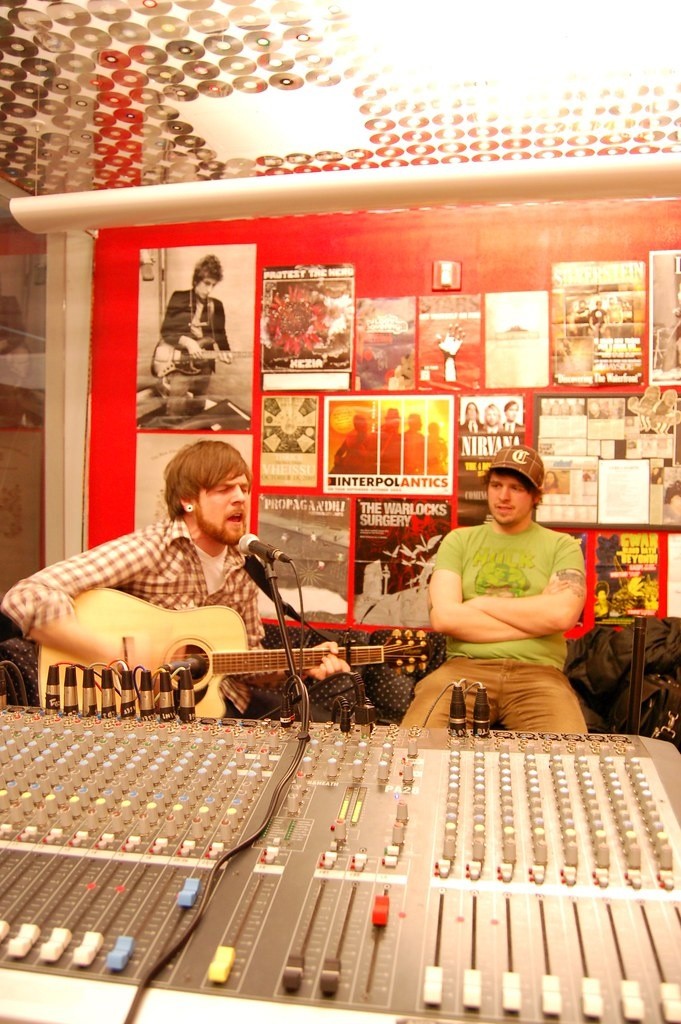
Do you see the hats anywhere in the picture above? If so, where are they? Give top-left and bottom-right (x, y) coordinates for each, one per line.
(491, 446), (544, 490)
(385, 408), (401, 419)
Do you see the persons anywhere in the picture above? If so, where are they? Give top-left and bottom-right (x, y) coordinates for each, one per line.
(0, 441), (353, 723)
(398, 445), (589, 736)
(460, 402), (526, 434)
(542, 470), (560, 494)
(329, 410), (448, 475)
(161, 253), (234, 418)
(435, 322), (466, 384)
(573, 296), (633, 339)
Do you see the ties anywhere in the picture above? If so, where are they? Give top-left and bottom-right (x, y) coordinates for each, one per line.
(472, 423), (474, 432)
(196, 296), (215, 374)
(508, 426), (510, 442)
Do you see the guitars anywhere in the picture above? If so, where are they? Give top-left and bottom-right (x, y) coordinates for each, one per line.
(148, 331), (254, 379)
(34, 585), (435, 722)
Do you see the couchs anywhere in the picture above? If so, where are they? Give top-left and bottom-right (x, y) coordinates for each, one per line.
(0, 613), (681, 751)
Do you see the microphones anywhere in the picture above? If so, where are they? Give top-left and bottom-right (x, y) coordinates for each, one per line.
(238, 534), (292, 564)
